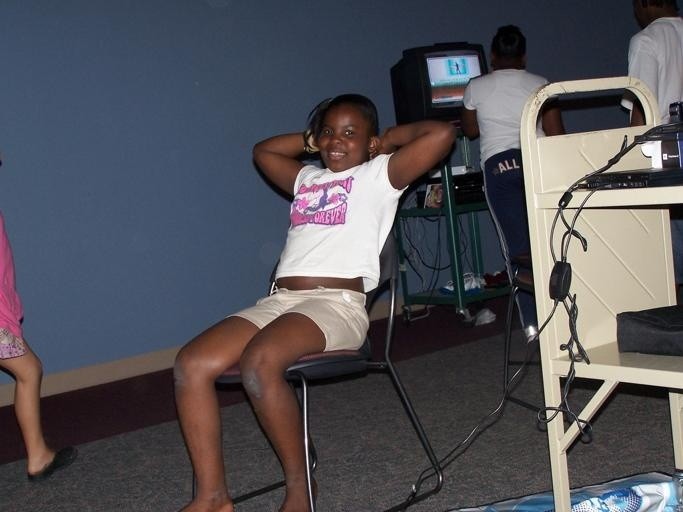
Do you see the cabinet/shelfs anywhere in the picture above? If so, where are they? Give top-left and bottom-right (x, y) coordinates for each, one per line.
(518, 72), (683, 512)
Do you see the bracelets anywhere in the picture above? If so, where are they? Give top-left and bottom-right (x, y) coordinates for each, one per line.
(302, 129), (319, 154)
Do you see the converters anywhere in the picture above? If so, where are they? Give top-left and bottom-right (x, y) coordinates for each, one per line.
(548, 260), (571, 302)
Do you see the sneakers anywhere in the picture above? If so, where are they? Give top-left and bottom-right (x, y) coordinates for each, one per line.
(525, 327), (540, 343)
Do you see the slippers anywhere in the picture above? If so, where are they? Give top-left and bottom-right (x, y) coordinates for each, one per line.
(27, 446), (78, 481)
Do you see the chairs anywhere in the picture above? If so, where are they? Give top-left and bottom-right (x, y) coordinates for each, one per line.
(209, 228), (444, 512)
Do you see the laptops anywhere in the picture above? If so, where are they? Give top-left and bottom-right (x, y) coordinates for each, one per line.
(587, 168), (681, 189)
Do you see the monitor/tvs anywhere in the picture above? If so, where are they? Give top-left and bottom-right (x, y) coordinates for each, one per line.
(389, 42), (489, 125)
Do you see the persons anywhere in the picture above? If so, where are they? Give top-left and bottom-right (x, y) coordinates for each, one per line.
(175, 94), (456, 512)
(461, 25), (565, 344)
(0, 204), (78, 482)
(621, 2), (682, 127)
